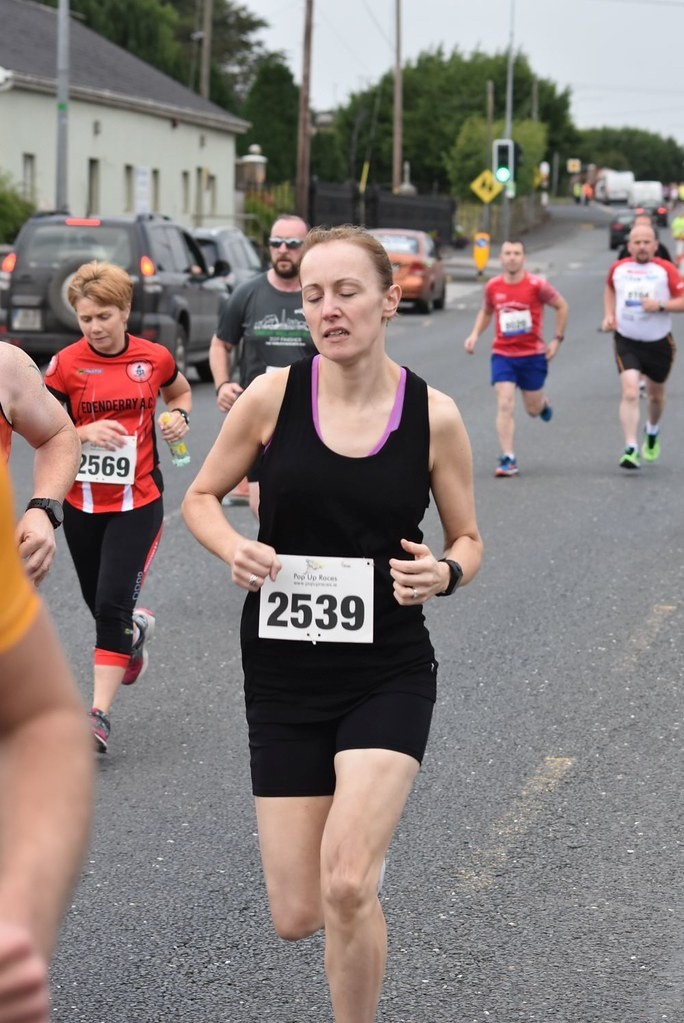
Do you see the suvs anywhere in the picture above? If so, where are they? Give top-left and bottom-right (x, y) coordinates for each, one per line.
(1, 210), (234, 395)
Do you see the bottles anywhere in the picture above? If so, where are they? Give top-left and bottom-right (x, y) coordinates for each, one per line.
(162, 411), (191, 468)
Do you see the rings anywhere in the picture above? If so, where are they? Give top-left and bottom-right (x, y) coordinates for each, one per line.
(248, 574), (258, 584)
(411, 588), (418, 600)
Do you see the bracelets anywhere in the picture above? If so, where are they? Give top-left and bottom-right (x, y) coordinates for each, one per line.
(553, 335), (564, 342)
(216, 381), (230, 396)
(172, 408), (190, 424)
(659, 301), (664, 311)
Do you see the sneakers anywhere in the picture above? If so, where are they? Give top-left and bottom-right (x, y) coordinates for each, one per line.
(494, 457), (519, 476)
(85, 708), (111, 753)
(619, 447), (641, 469)
(540, 397), (553, 422)
(122, 607), (155, 685)
(642, 423), (661, 461)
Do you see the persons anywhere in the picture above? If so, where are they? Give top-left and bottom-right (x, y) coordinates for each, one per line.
(0, 341), (81, 587)
(209, 215), (320, 519)
(601, 211), (684, 469)
(180, 224), (481, 1023)
(0, 450), (96, 1023)
(464, 240), (569, 476)
(45, 261), (192, 754)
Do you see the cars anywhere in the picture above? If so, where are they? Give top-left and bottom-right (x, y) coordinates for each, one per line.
(631, 200), (668, 227)
(191, 227), (272, 292)
(366, 231), (447, 314)
(609, 211), (658, 250)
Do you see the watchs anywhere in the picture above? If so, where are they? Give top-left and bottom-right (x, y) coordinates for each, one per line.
(435, 558), (463, 597)
(25, 498), (64, 529)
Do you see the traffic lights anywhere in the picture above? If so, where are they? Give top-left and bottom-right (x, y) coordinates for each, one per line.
(493, 140), (513, 184)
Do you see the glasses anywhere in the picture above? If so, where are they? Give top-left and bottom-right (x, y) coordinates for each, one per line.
(267, 237), (306, 249)
